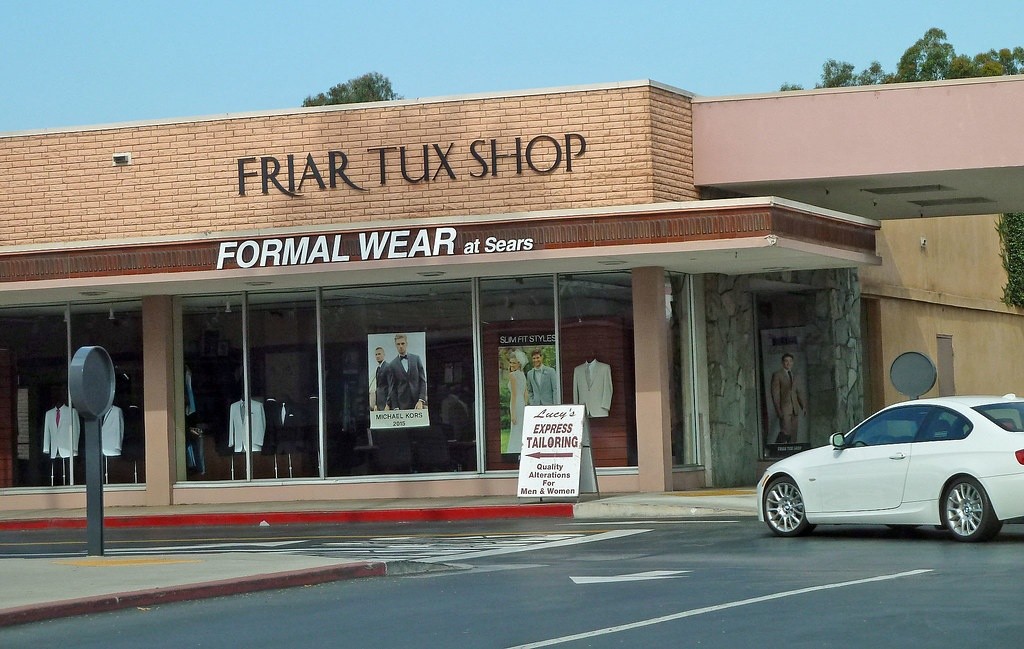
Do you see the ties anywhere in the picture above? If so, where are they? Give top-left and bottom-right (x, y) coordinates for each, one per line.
(240, 403), (245, 423)
(788, 370), (794, 382)
(281, 403), (285, 424)
(102, 414), (106, 425)
(56, 409), (60, 427)
(586, 364), (592, 387)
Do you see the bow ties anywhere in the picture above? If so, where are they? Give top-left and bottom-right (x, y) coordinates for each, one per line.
(400, 356), (407, 360)
(535, 367), (542, 373)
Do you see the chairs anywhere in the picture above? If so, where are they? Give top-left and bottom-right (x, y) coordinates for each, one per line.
(927, 420), (950, 439)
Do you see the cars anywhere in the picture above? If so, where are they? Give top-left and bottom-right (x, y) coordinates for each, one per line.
(753, 396), (1024, 538)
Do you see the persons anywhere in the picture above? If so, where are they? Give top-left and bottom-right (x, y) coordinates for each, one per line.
(508, 350), (529, 452)
(373, 346), (390, 411)
(527, 348), (559, 405)
(771, 353), (806, 443)
(389, 334), (426, 410)
